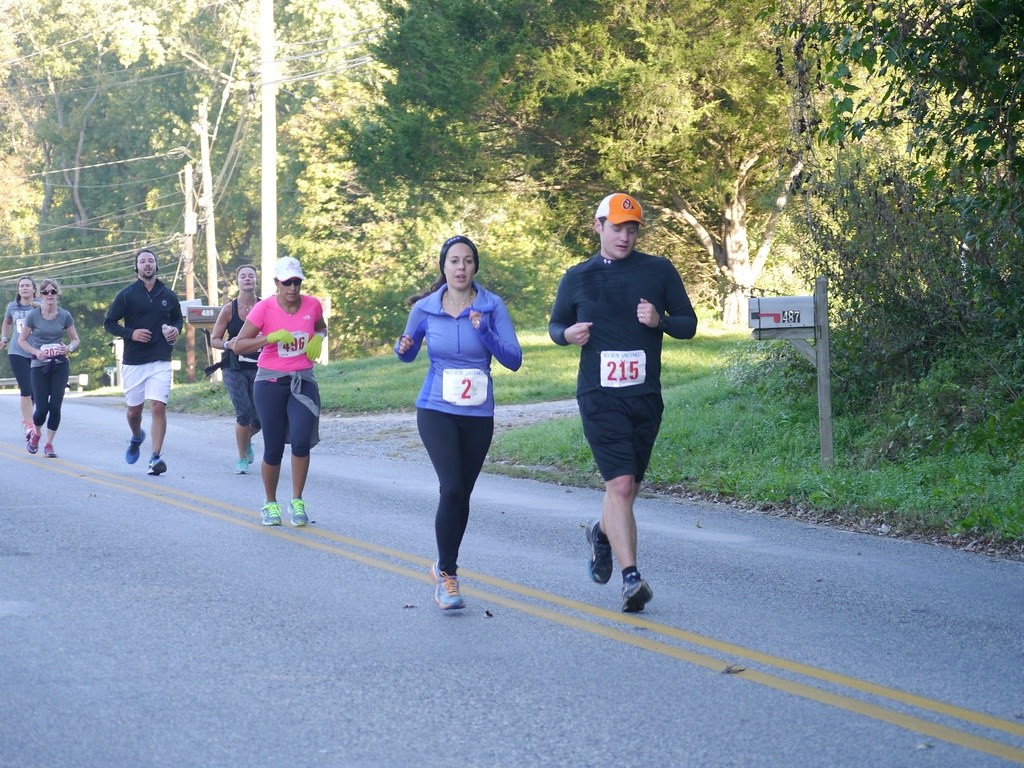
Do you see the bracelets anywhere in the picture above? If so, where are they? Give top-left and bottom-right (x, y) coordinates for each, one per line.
(67, 344), (75, 354)
(2, 336), (7, 342)
(223, 341), (229, 350)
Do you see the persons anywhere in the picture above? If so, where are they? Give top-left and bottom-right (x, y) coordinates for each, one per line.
(0, 276), (41, 441)
(549, 191), (698, 612)
(393, 236), (522, 610)
(104, 249), (183, 476)
(18, 279), (80, 457)
(233, 255), (327, 527)
(210, 264), (264, 474)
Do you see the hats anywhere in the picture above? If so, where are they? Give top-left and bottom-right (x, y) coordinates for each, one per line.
(274, 256), (306, 282)
(440, 236), (479, 275)
(596, 193), (645, 227)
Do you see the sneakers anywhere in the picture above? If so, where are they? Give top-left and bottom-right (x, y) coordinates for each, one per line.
(585, 519), (613, 584)
(433, 558), (464, 610)
(622, 571), (652, 612)
(247, 439), (254, 464)
(261, 499), (282, 526)
(288, 496), (308, 527)
(235, 457), (248, 474)
(147, 452), (166, 475)
(27, 430), (41, 454)
(44, 443), (57, 458)
(126, 429), (146, 464)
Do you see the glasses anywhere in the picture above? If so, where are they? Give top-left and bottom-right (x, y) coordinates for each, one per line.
(42, 289), (58, 295)
(281, 280), (302, 286)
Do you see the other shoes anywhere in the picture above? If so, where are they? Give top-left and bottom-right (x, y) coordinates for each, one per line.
(25, 425), (33, 441)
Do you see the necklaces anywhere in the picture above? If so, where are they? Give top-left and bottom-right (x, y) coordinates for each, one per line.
(442, 287), (474, 310)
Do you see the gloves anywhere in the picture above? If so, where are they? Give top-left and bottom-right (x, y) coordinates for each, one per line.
(267, 329), (294, 346)
(307, 335), (322, 361)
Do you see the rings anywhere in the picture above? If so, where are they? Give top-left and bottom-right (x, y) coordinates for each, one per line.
(477, 320), (479, 324)
(641, 313), (644, 318)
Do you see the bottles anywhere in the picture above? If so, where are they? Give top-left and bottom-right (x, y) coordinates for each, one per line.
(2, 337), (7, 349)
(162, 324), (177, 345)
